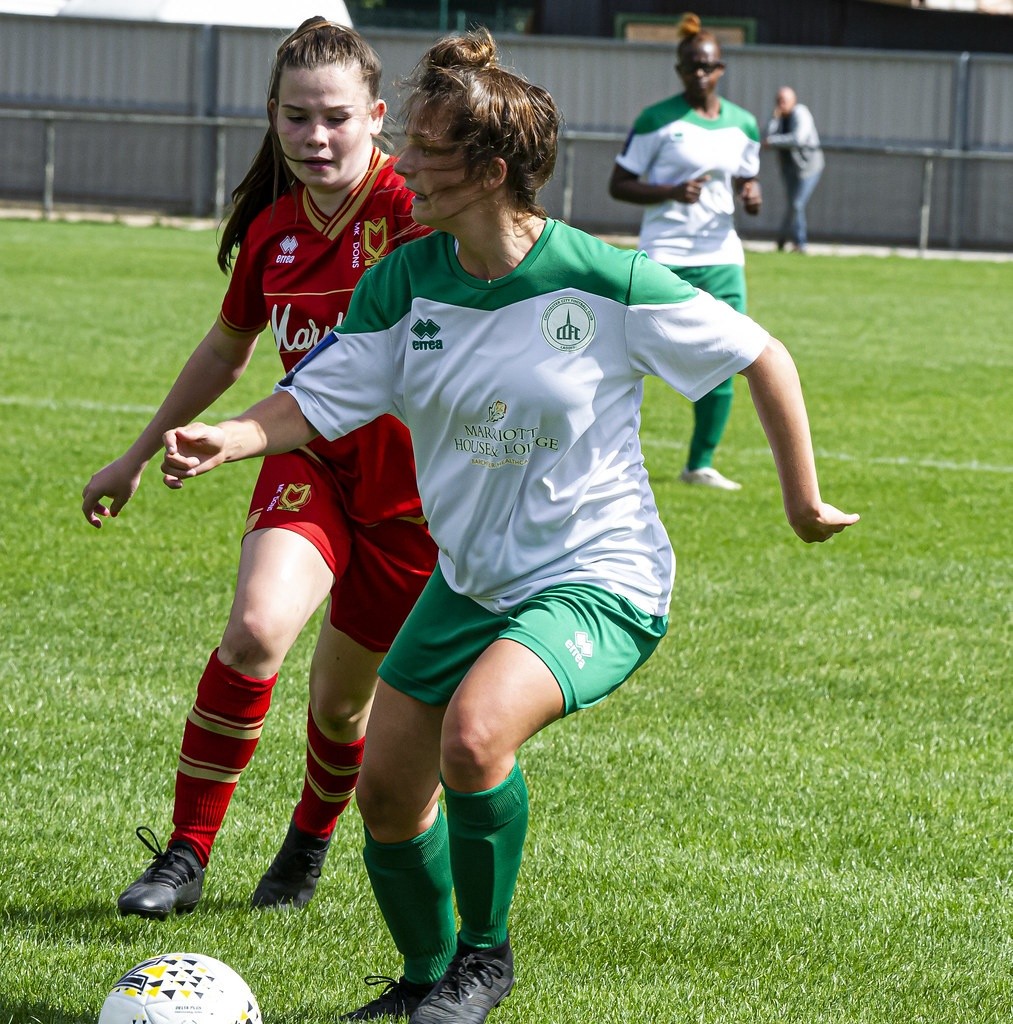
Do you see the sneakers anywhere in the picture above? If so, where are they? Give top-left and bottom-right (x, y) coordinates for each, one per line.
(411, 933), (514, 1022)
(250, 807), (333, 912)
(681, 466), (743, 492)
(337, 977), (434, 1020)
(119, 841), (204, 919)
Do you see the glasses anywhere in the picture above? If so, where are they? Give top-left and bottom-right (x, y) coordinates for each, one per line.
(679, 60), (723, 72)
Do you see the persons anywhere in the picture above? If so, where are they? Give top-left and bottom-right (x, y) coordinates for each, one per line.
(608, 12), (763, 490)
(82, 15), (444, 919)
(764, 85), (825, 255)
(156, 34), (858, 1024)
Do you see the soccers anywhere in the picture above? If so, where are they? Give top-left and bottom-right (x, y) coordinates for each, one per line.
(97, 954), (264, 1022)
